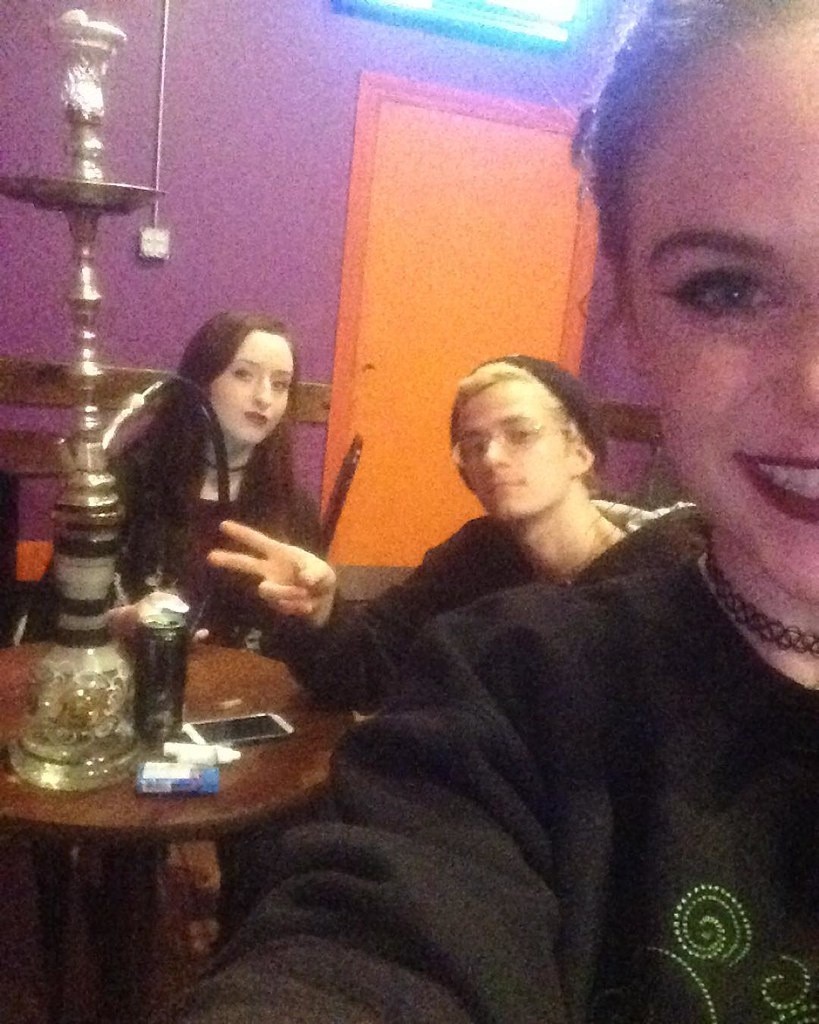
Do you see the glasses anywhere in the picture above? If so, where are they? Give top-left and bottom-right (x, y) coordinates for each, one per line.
(450, 422), (571, 470)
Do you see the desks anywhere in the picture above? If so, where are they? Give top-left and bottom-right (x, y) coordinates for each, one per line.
(0, 633), (360, 1024)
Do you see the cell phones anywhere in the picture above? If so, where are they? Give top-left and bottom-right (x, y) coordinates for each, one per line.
(181, 712), (295, 747)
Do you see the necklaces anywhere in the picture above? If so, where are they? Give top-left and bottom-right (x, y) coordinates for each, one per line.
(199, 456), (246, 473)
(702, 532), (819, 658)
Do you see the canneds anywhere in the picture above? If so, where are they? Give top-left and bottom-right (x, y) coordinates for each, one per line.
(134, 615), (186, 746)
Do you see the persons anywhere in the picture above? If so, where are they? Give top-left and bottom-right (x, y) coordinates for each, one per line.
(186, 0), (819, 1024)
(18, 309), (323, 664)
(205, 356), (697, 718)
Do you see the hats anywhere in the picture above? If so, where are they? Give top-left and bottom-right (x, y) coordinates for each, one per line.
(451, 355), (608, 469)
